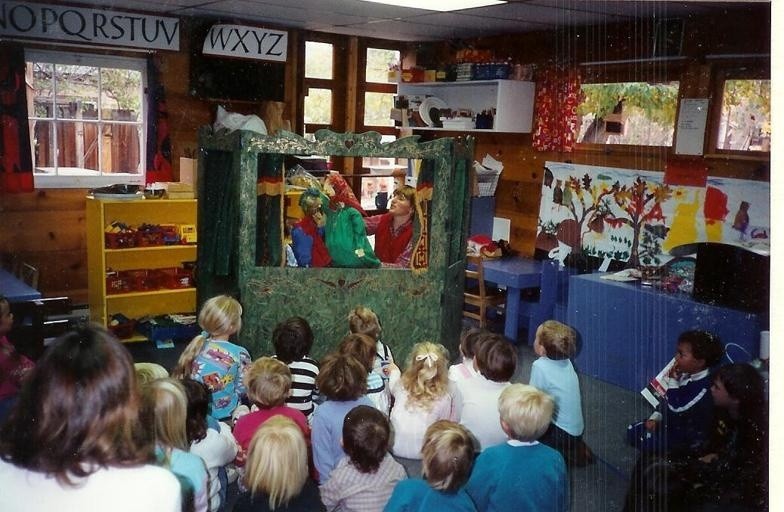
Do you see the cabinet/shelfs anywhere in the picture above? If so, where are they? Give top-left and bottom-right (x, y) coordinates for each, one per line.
(85, 195), (200, 346)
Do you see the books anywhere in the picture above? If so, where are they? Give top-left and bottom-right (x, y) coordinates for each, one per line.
(455, 62), (477, 82)
(439, 117), (477, 129)
(144, 180), (195, 200)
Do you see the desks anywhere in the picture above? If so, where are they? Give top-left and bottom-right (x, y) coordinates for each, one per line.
(564, 269), (760, 401)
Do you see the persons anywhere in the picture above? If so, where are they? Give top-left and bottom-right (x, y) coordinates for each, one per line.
(0, 292), (528, 466)
(387, 413), (477, 510)
(462, 381), (576, 512)
(121, 360), (210, 511)
(155, 373), (240, 512)
(624, 454), (690, 512)
(361, 184), (422, 268)
(318, 402), (407, 510)
(226, 414), (317, 509)
(530, 318), (592, 464)
(628, 330), (724, 458)
(698, 362), (769, 511)
(1, 327), (184, 510)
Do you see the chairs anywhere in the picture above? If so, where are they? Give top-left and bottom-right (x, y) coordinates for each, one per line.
(462, 257), (576, 349)
(1, 259), (71, 363)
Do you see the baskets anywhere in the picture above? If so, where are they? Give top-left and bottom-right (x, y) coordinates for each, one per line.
(109, 312), (200, 340)
(104, 232), (165, 248)
(106, 267), (195, 295)
